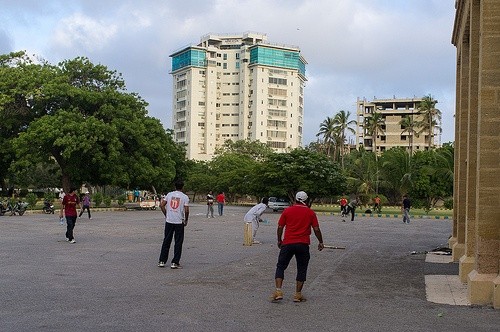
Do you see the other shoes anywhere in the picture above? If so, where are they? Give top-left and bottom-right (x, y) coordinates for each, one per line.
(70, 239), (76, 244)
(65, 237), (69, 241)
(252, 240), (259, 244)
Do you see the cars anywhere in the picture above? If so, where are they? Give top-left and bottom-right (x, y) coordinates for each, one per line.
(267, 196), (291, 212)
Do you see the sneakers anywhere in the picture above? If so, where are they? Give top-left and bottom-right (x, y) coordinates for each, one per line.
(270, 291), (283, 302)
(294, 294), (306, 302)
(171, 263), (182, 269)
(157, 262), (165, 266)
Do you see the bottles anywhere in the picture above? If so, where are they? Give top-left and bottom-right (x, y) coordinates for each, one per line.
(60, 216), (63, 225)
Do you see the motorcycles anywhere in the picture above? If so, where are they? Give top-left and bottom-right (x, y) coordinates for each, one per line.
(0, 196), (29, 215)
(43, 196), (55, 214)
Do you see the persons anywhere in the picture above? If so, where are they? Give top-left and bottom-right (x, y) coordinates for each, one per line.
(340, 197), (359, 221)
(374, 196), (382, 212)
(244, 197), (268, 245)
(403, 196), (411, 224)
(78, 193), (93, 220)
(133, 188), (139, 202)
(158, 181), (190, 268)
(216, 191), (225, 216)
(60, 187), (83, 243)
(59, 188), (65, 209)
(161, 193), (165, 205)
(207, 190), (214, 218)
(273, 191), (324, 301)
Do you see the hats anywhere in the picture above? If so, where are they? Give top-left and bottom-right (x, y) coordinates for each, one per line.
(296, 191), (308, 205)
